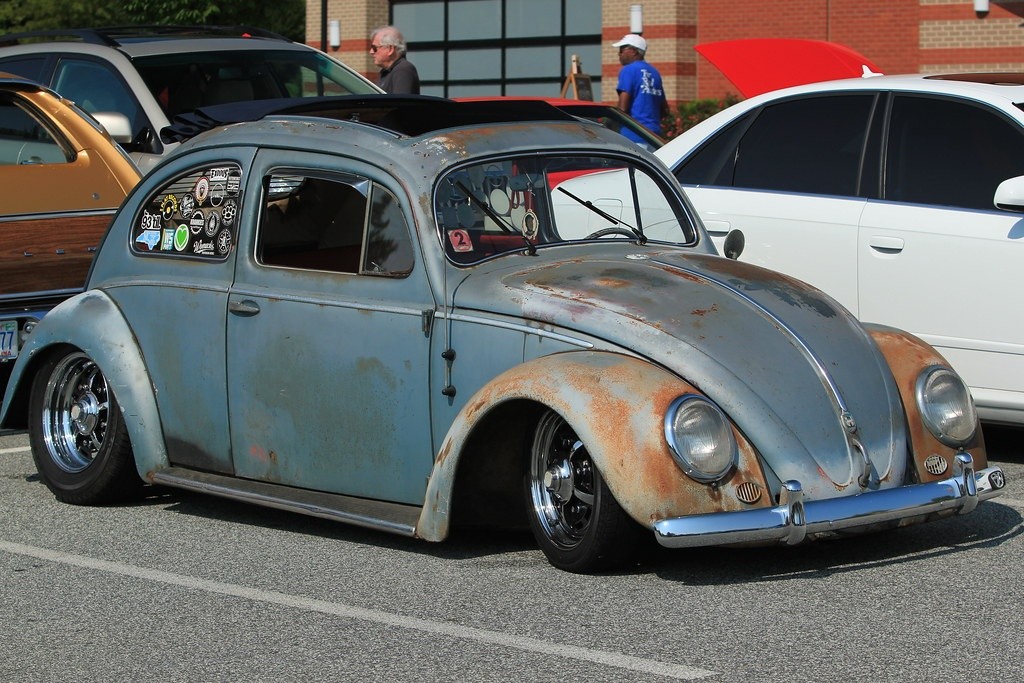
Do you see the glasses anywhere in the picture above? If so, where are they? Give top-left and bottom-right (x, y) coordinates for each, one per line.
(370, 44), (386, 53)
(620, 46), (631, 53)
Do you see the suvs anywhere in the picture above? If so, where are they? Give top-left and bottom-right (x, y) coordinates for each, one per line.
(0, 28), (387, 363)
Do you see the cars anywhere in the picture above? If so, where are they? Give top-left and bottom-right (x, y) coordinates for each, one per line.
(450, 38), (1024, 427)
(0, 94), (1007, 575)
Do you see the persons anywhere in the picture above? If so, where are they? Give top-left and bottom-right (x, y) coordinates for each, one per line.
(611, 34), (671, 154)
(525, 215), (535, 236)
(369, 25), (420, 95)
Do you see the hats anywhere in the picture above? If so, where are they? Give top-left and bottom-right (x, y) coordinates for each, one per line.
(612, 35), (647, 51)
